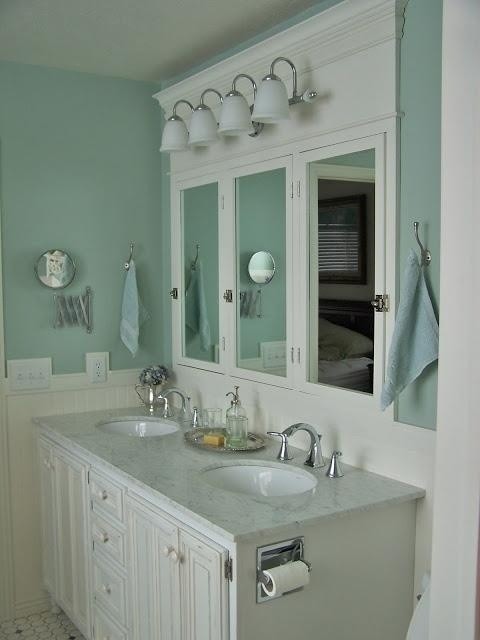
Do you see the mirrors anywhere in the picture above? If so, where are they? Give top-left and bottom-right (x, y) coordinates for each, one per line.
(248, 252), (276, 284)
(35, 249), (77, 289)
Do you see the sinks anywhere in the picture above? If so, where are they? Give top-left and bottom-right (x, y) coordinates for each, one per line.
(95, 416), (181, 438)
(198, 459), (318, 498)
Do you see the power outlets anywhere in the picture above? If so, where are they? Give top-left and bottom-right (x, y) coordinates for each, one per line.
(85, 351), (110, 388)
(260, 341), (289, 371)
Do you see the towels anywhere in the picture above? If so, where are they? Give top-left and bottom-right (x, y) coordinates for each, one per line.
(184, 260), (211, 352)
(379, 250), (440, 415)
(120, 256), (152, 359)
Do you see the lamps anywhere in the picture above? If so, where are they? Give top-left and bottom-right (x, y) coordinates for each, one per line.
(158, 56), (317, 154)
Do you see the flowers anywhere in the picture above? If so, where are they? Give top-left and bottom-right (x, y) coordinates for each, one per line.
(140, 366), (168, 389)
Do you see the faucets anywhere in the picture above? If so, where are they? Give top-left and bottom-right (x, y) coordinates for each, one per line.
(283, 422), (325, 469)
(155, 387), (193, 422)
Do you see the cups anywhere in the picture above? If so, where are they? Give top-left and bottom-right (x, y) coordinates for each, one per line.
(202, 407), (223, 436)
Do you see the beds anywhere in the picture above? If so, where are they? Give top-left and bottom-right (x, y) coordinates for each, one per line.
(318, 300), (376, 395)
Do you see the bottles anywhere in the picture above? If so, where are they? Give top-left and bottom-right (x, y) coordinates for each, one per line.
(225, 398), (249, 450)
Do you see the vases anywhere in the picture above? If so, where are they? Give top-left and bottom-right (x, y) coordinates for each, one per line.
(147, 382), (157, 406)
